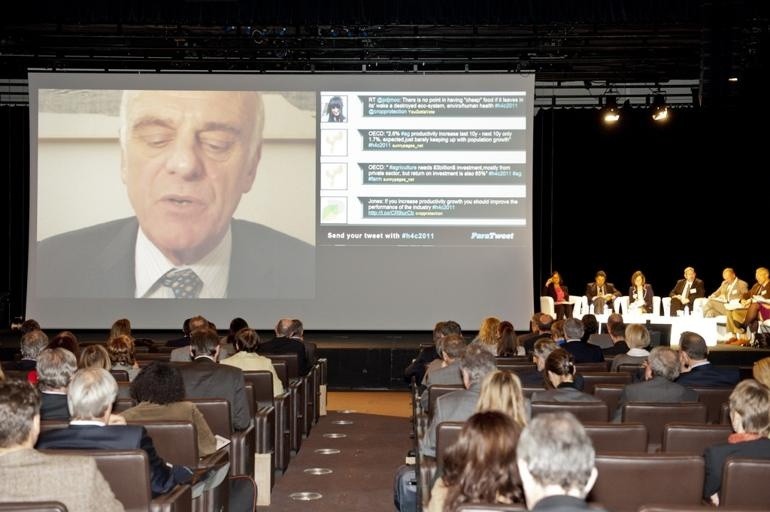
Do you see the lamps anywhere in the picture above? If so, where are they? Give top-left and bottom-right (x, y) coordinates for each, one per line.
(600, 90), (622, 125)
(651, 93), (670, 123)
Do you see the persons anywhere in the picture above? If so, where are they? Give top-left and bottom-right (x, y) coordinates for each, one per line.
(542, 267), (769, 342)
(35, 90), (315, 300)
(1, 316), (315, 511)
(404, 314), (770, 512)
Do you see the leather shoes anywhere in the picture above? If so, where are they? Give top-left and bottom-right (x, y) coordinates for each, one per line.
(725, 337), (738, 344)
(744, 339), (759, 349)
(734, 321), (747, 333)
(731, 339), (751, 347)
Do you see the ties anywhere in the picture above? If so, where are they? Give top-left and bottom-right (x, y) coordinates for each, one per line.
(600, 286), (602, 292)
(686, 285), (691, 299)
(160, 269), (203, 299)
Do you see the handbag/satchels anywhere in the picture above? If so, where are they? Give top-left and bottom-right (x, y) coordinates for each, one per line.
(393, 464), (418, 512)
